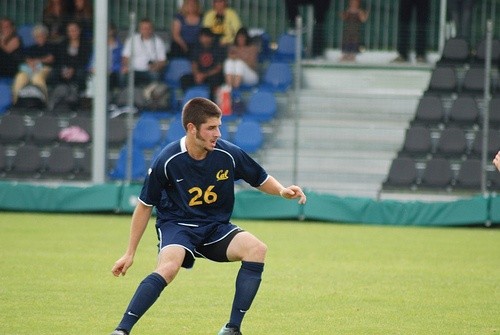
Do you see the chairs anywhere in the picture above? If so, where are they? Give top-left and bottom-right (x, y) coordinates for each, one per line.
(382, 37), (500, 192)
(0, 23), (307, 180)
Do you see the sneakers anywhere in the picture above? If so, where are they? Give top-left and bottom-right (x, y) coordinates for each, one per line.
(111, 330), (127, 335)
(218, 323), (242, 335)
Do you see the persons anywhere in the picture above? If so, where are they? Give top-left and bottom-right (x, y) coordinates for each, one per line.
(110, 97), (306, 335)
(340, 0), (370, 62)
(0, 0), (332, 112)
(392, 0), (430, 63)
(493, 151), (500, 172)
(448, 0), (473, 46)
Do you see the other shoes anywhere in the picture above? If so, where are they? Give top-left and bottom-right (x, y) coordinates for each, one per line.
(416, 58), (428, 66)
(393, 56), (410, 63)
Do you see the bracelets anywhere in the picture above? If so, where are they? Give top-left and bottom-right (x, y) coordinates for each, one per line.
(279, 189), (289, 199)
